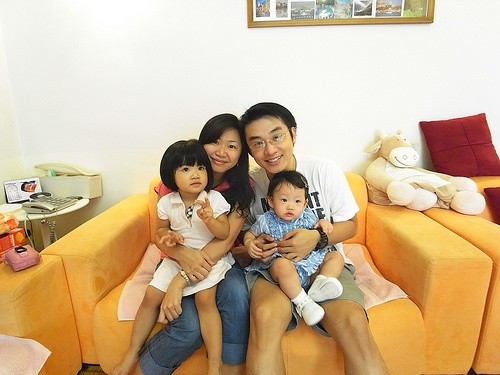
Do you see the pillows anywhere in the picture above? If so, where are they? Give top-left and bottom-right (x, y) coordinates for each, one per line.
(419, 112), (500, 178)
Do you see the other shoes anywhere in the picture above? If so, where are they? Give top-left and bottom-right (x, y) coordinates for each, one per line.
(295, 296), (325, 327)
(308, 277), (344, 303)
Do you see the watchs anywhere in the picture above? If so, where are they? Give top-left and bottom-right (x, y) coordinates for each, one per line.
(308, 228), (328, 251)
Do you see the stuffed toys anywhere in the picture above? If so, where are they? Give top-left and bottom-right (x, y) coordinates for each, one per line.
(363, 134), (486, 215)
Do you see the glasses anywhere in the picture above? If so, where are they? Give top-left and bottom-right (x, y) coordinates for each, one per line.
(249, 130), (289, 153)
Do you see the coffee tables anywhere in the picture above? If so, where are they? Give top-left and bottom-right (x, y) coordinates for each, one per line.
(0, 196), (90, 248)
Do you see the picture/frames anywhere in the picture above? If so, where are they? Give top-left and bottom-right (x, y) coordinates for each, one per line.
(247, 0), (435, 28)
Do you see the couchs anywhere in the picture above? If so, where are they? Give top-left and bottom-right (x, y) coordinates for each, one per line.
(0, 169), (500, 375)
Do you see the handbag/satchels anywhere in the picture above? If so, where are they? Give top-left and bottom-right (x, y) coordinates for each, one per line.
(5, 245), (41, 272)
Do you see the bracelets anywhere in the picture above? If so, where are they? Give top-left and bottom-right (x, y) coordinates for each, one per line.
(180, 270), (191, 285)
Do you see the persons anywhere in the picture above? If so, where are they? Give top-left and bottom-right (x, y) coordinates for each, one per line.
(111, 138), (231, 375)
(21, 182), (37, 191)
(134, 113), (256, 375)
(243, 170), (346, 326)
(241, 102), (391, 375)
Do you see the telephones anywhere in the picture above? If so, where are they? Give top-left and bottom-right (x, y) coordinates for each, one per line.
(21, 196), (78, 214)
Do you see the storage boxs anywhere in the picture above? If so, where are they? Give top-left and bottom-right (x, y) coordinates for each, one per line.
(37, 175), (103, 200)
(0, 227), (26, 255)
